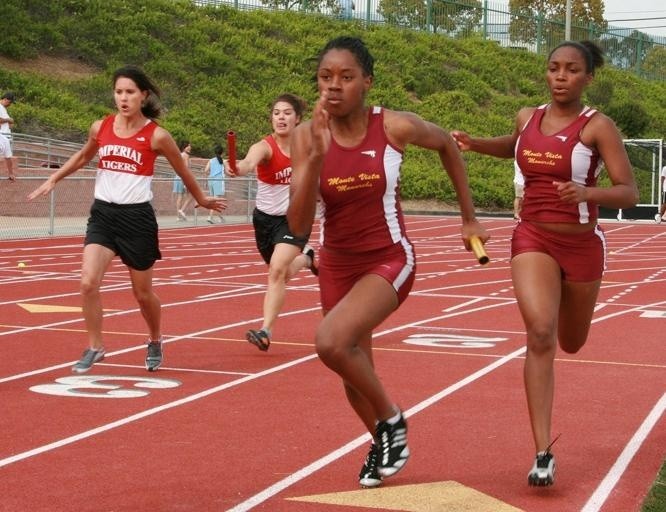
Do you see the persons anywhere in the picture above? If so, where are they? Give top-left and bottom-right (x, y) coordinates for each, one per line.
(0, 93), (16, 181)
(172, 140), (193, 222)
(660, 166), (666, 217)
(27, 67), (227, 374)
(287, 37), (489, 486)
(510, 153), (524, 222)
(224, 93), (319, 351)
(204, 145), (226, 224)
(450, 38), (638, 488)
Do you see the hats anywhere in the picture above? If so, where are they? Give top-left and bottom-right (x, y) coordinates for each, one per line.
(5, 92), (17, 104)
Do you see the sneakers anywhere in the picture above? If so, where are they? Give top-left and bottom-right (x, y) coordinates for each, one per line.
(72, 346), (106, 374)
(206, 217), (216, 224)
(178, 209), (188, 222)
(177, 219), (187, 223)
(303, 245), (319, 278)
(359, 443), (386, 489)
(217, 218), (225, 224)
(245, 329), (271, 352)
(528, 451), (558, 487)
(144, 337), (163, 372)
(375, 408), (411, 478)
